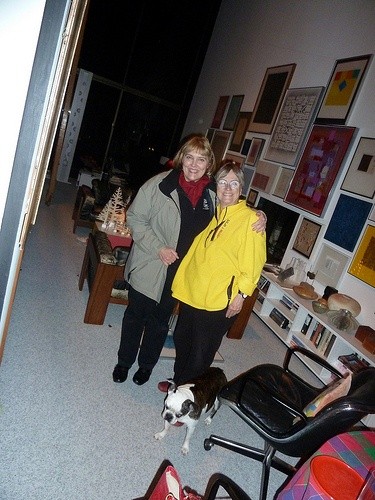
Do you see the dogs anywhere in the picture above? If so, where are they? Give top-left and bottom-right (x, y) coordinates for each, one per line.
(153, 366), (228, 457)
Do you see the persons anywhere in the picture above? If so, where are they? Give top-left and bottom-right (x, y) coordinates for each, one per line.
(112, 136), (267, 386)
(158, 159), (268, 393)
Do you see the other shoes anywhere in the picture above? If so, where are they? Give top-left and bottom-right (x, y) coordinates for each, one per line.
(132, 369), (152, 385)
(158, 381), (172, 392)
(113, 364), (129, 383)
(176, 421), (184, 426)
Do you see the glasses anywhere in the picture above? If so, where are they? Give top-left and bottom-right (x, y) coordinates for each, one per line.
(217, 179), (241, 189)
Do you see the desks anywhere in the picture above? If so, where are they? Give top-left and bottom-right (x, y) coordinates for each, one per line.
(95, 220), (132, 249)
(275, 430), (375, 500)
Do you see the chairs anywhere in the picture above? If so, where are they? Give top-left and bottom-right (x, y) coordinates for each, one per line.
(204, 345), (375, 500)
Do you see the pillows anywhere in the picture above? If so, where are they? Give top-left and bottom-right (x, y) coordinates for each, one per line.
(303, 371), (352, 417)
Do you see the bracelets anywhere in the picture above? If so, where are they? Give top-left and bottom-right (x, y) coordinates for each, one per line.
(257, 208), (267, 224)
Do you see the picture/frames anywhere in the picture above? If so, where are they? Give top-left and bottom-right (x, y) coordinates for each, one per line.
(204, 52), (375, 290)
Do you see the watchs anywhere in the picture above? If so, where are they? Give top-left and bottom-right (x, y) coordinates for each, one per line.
(238, 290), (248, 299)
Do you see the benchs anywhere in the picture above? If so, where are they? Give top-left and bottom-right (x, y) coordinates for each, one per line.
(73, 179), (119, 234)
(77, 232), (259, 340)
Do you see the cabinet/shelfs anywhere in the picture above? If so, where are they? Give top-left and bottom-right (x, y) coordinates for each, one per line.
(252, 271), (375, 387)
